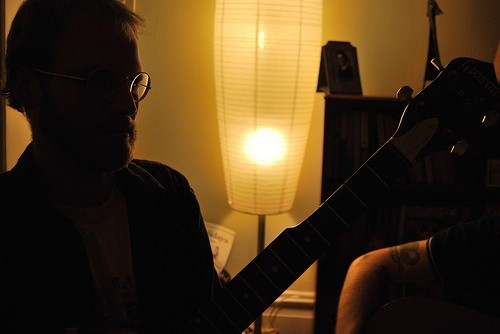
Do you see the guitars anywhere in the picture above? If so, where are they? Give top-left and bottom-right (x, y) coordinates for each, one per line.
(176, 54), (500, 334)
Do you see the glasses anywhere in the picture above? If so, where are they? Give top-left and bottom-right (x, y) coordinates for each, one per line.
(33, 69), (152, 103)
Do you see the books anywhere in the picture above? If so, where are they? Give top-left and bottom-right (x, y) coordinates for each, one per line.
(331, 107), (500, 304)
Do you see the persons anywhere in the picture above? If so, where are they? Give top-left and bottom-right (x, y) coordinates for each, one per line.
(0, 0), (223, 334)
(337, 51), (355, 82)
(336, 212), (500, 334)
